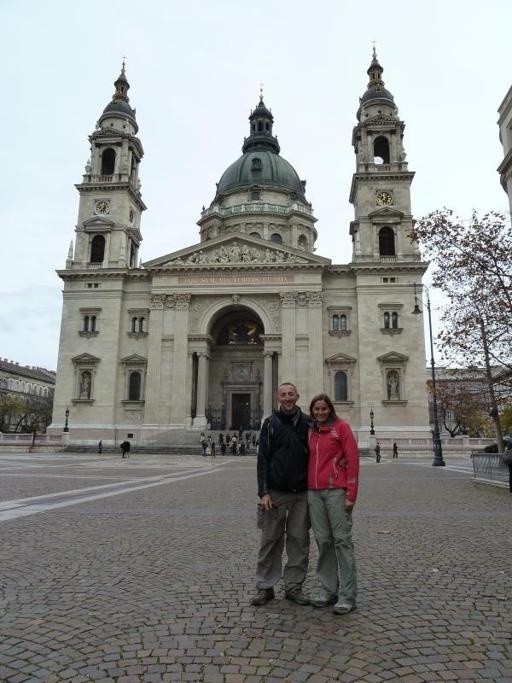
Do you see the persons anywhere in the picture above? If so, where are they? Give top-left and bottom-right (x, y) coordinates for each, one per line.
(305, 392), (360, 614)
(392, 441), (400, 458)
(120, 439), (126, 458)
(374, 441), (382, 463)
(126, 440), (131, 458)
(200, 422), (259, 457)
(98, 438), (103, 452)
(249, 381), (348, 607)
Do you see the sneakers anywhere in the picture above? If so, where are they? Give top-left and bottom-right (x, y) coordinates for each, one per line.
(311, 594), (339, 608)
(252, 589), (275, 606)
(333, 600), (353, 615)
(285, 587), (310, 605)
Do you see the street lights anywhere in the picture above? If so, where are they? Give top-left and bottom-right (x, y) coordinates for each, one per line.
(369, 407), (375, 434)
(63, 407), (69, 432)
(411, 297), (446, 467)
